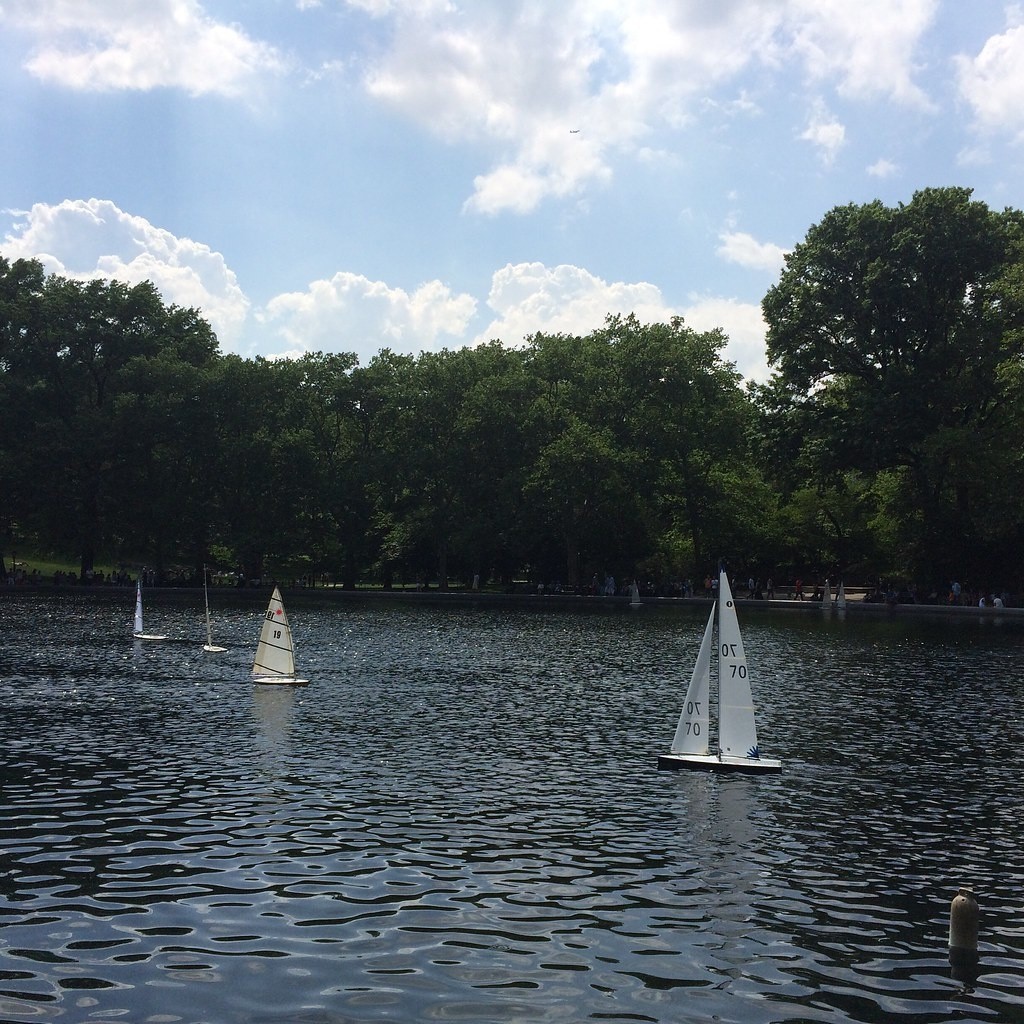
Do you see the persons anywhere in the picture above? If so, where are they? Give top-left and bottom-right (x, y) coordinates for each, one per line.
(7, 568), (1024, 608)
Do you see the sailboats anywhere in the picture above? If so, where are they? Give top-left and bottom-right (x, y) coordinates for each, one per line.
(131, 580), (169, 639)
(819, 579), (831, 610)
(657, 569), (782, 774)
(837, 582), (847, 610)
(248, 585), (309, 685)
(203, 563), (228, 652)
(628, 578), (644, 604)
